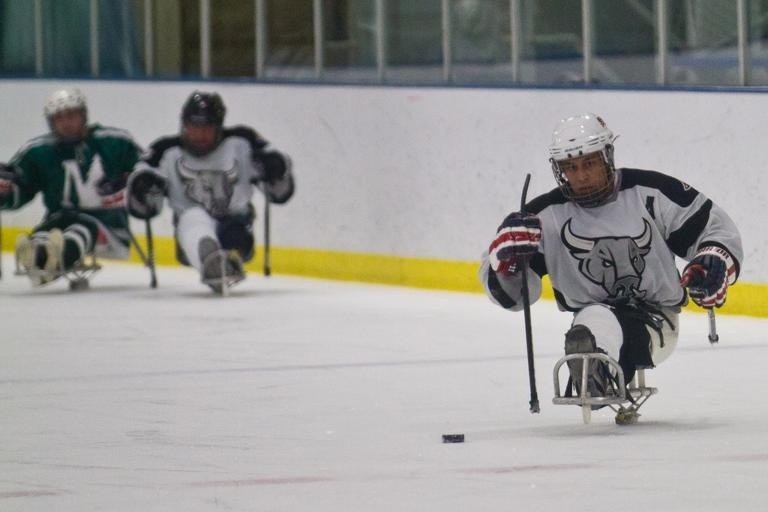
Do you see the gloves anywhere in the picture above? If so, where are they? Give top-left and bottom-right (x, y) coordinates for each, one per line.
(260, 152), (286, 180)
(488, 211), (542, 275)
(97, 175), (128, 210)
(0, 164), (23, 200)
(131, 172), (165, 205)
(678, 254), (729, 308)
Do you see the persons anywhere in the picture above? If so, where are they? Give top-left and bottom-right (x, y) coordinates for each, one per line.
(123, 90), (295, 294)
(477, 111), (745, 411)
(1, 88), (146, 282)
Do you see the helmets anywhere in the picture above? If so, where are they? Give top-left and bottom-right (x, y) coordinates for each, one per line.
(180, 92), (225, 155)
(549, 111), (619, 208)
(42, 90), (87, 144)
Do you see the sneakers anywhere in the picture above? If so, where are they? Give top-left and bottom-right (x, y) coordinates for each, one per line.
(17, 228), (63, 286)
(199, 236), (222, 294)
(565, 325), (609, 410)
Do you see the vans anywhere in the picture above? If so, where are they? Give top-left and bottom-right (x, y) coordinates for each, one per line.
(262, 31), (614, 83)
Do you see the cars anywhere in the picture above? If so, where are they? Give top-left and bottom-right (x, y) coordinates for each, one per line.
(661, 11), (766, 88)
